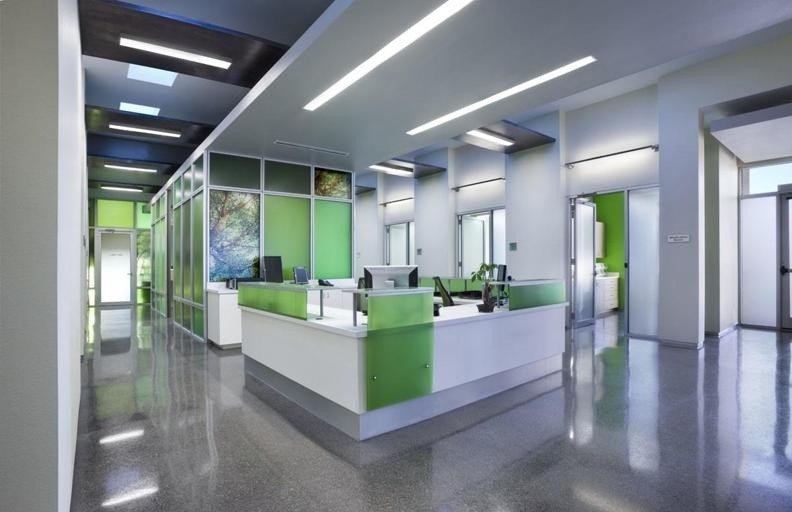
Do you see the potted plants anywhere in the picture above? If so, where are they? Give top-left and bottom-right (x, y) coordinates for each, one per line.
(470, 262), (497, 302)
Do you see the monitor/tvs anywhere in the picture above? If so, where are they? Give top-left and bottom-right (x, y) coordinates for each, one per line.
(293, 266), (309, 284)
(363, 265), (419, 298)
(497, 264), (506, 291)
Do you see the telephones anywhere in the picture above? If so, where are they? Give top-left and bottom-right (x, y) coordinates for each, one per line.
(319, 279), (334, 286)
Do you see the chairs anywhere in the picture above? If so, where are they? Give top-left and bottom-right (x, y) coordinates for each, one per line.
(432, 276), (455, 307)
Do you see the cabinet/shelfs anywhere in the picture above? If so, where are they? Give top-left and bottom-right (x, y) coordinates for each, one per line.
(595, 279), (620, 312)
